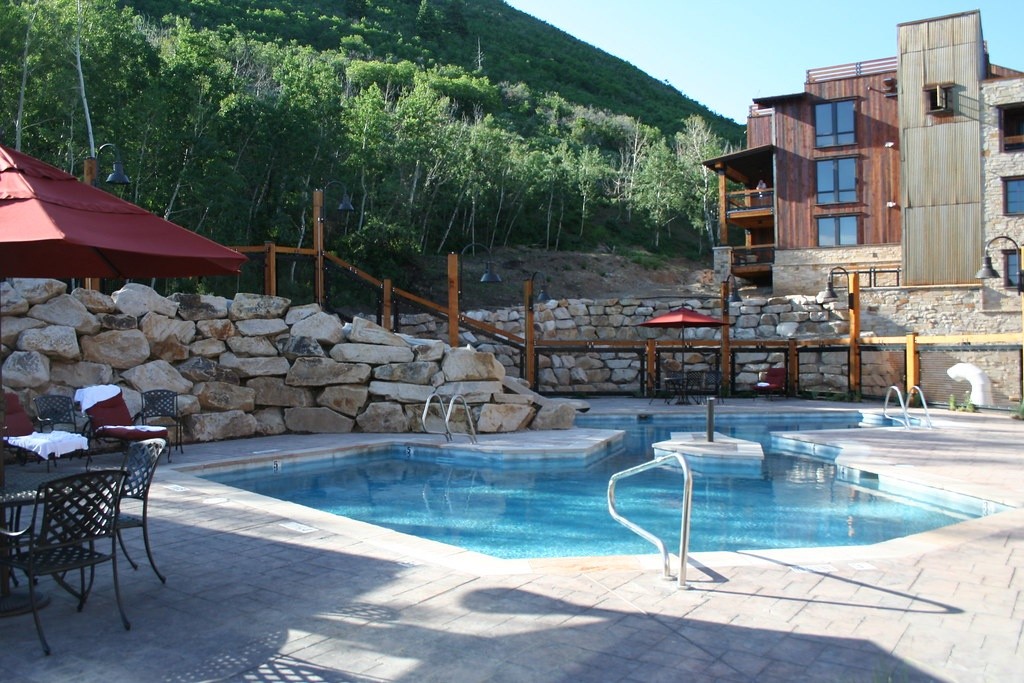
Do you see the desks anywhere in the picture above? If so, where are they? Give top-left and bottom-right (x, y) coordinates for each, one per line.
(664, 377), (703, 405)
(0, 471), (103, 618)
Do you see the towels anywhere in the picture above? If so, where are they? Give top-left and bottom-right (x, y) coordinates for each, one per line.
(3, 430), (89, 460)
(95, 425), (167, 433)
(73, 384), (120, 414)
(757, 382), (770, 387)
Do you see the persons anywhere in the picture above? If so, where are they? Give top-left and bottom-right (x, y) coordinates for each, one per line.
(756, 180), (767, 206)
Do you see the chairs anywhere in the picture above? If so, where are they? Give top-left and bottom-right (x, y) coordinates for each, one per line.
(140, 389), (184, 455)
(647, 370), (724, 404)
(751, 367), (789, 401)
(73, 383), (167, 464)
(33, 395), (92, 467)
(0, 470), (131, 657)
(61, 438), (167, 585)
(5, 393), (89, 472)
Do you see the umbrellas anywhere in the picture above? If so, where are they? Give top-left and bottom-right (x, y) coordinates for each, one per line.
(631, 303), (732, 401)
(0, 144), (249, 281)
